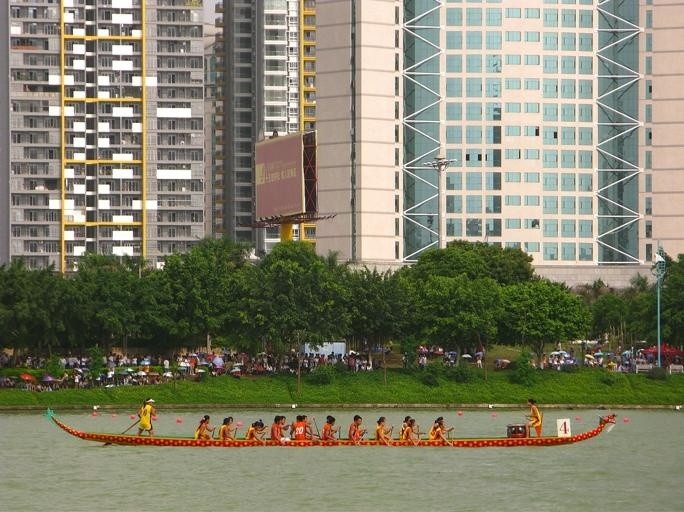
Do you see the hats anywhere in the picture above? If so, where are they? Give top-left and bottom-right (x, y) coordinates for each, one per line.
(146, 399), (156, 403)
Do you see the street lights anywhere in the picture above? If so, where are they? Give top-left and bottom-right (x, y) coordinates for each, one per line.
(649, 253), (664, 369)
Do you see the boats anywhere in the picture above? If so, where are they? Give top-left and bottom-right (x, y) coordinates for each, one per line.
(46, 410), (619, 452)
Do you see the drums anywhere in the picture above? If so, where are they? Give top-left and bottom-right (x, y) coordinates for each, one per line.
(506, 423), (531, 439)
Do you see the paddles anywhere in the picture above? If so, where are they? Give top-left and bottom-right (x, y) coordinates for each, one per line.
(104, 418), (140, 445)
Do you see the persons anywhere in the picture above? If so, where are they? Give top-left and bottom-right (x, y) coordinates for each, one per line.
(401, 346), (486, 368)
(195, 414), (457, 448)
(136, 396), (158, 438)
(495, 340), (683, 372)
(2, 345), (391, 394)
(523, 398), (543, 438)
